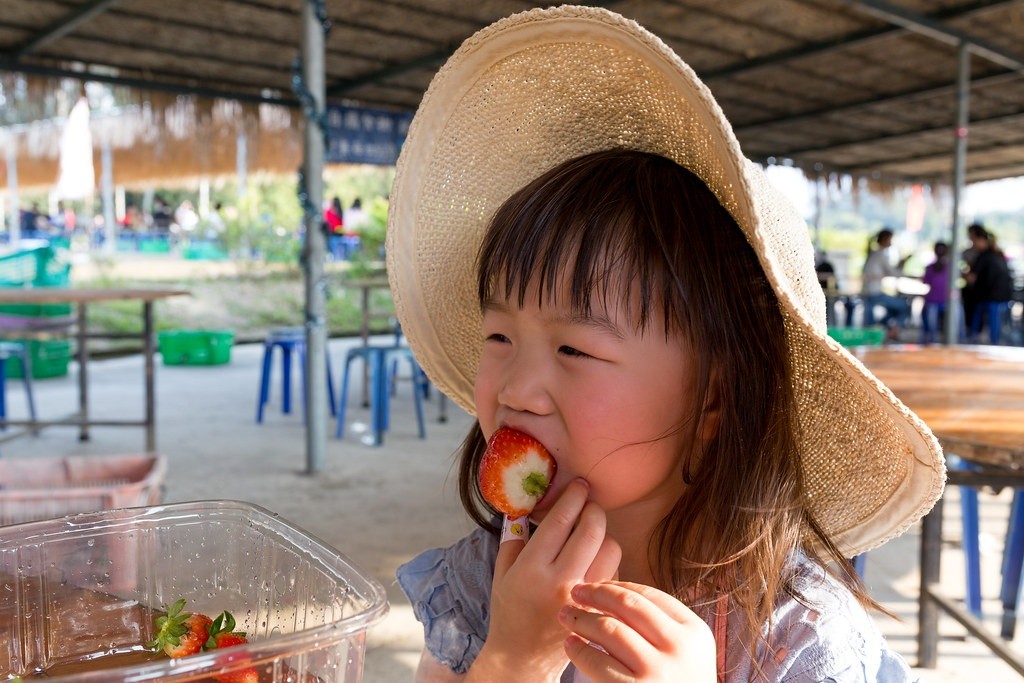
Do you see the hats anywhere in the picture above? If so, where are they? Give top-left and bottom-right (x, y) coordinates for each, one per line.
(386, 4), (947, 565)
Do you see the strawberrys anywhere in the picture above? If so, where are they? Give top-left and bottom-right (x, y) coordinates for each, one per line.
(146, 599), (214, 658)
(479, 426), (555, 518)
(204, 611), (258, 683)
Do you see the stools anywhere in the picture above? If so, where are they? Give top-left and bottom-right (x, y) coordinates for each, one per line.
(919, 304), (967, 347)
(337, 347), (427, 451)
(391, 320), (430, 402)
(0, 341), (39, 438)
(255, 326), (337, 427)
(973, 300), (1015, 346)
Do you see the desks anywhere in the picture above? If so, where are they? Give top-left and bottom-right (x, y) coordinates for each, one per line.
(0, 288), (193, 449)
(858, 343), (1024, 678)
(345, 279), (449, 425)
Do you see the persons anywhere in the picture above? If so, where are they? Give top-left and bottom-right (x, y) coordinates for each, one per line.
(23, 195), (366, 235)
(817, 218), (1012, 338)
(383, 3), (946, 683)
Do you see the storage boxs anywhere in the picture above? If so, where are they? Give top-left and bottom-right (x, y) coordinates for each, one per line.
(152, 328), (236, 369)
(0, 498), (393, 683)
(0, 452), (169, 590)
(0, 237), (73, 378)
